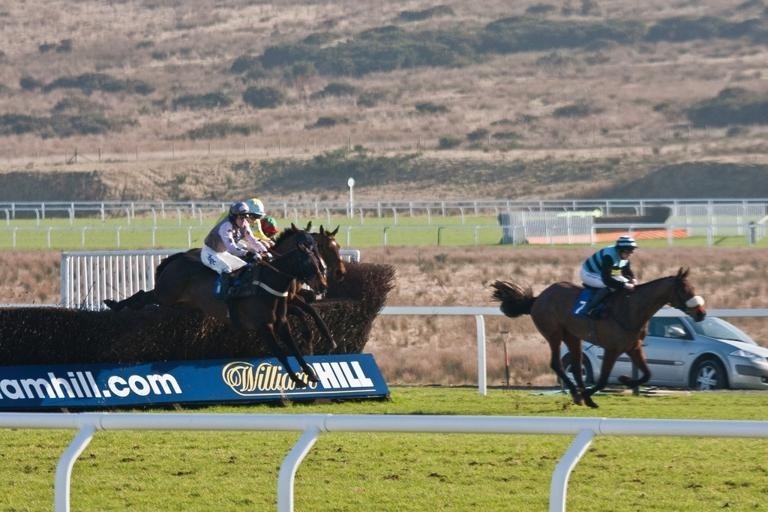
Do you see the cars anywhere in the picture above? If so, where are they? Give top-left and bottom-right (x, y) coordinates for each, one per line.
(553, 304), (768, 390)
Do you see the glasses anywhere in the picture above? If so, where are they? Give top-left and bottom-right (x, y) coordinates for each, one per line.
(240, 214), (262, 219)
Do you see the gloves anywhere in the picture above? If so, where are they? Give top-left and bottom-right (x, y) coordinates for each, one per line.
(624, 278), (638, 289)
(248, 251), (273, 262)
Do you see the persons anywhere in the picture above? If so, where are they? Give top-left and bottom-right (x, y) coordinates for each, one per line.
(217, 195), (274, 247)
(258, 213), (280, 240)
(199, 201), (267, 281)
(580, 235), (638, 320)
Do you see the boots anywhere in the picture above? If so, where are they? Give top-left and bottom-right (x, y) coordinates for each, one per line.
(582, 287), (610, 321)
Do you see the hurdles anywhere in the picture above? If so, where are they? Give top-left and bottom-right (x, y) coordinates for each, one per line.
(0, 260), (396, 412)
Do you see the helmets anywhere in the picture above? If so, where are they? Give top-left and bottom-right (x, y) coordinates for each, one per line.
(260, 215), (280, 235)
(616, 235), (637, 248)
(230, 198), (266, 215)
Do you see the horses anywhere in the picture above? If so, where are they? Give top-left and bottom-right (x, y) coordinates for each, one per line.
(488, 267), (707, 408)
(103, 220), (346, 388)
